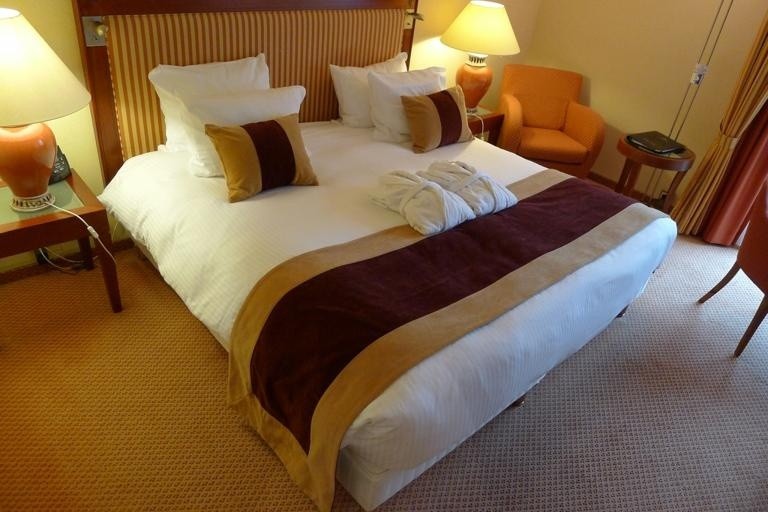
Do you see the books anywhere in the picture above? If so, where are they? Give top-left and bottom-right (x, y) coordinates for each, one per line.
(627, 132), (684, 157)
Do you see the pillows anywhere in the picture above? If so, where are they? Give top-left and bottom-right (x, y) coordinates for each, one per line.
(399, 84), (476, 154)
(144, 53), (271, 171)
(328, 51), (409, 131)
(367, 67), (448, 144)
(204, 113), (320, 205)
(172, 85), (308, 178)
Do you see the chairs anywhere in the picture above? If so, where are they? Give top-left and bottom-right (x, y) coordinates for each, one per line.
(698, 179), (768, 358)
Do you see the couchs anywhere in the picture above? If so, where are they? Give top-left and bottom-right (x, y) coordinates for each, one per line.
(497, 63), (607, 180)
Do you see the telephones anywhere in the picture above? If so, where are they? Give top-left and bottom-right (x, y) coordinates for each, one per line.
(47, 146), (71, 185)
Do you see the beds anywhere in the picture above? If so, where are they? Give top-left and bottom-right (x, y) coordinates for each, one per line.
(69, 0), (679, 512)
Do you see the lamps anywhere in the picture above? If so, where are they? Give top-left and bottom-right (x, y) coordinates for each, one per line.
(439, 1), (521, 117)
(0, 8), (93, 213)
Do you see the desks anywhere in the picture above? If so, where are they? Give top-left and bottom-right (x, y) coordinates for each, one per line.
(616, 132), (696, 215)
(0, 168), (124, 315)
(465, 103), (505, 147)
